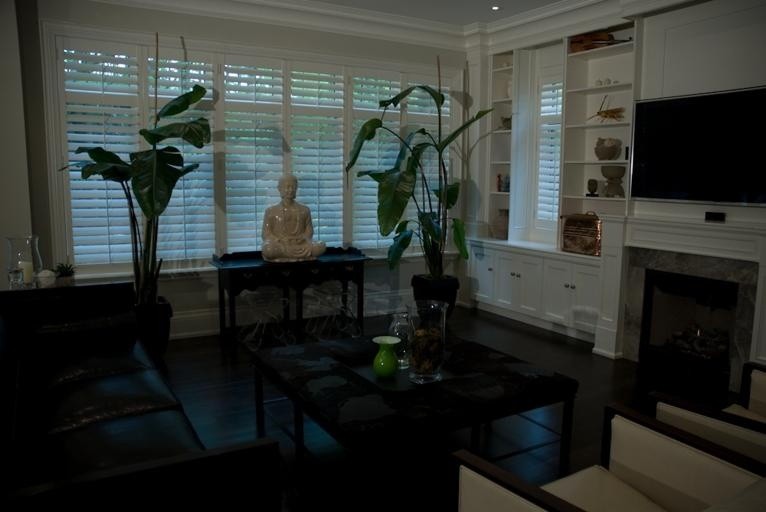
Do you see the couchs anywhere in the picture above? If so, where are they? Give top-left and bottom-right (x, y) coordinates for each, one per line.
(0, 313), (282, 512)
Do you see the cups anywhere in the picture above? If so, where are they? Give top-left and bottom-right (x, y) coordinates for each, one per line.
(405, 299), (448, 384)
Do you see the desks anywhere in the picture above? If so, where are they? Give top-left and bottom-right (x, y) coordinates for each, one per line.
(0, 280), (137, 360)
(208, 247), (374, 367)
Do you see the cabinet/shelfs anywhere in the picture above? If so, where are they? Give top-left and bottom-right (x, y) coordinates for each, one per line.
(468, 19), (644, 358)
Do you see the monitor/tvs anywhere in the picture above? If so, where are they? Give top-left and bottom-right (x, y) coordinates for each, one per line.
(628, 85), (766, 208)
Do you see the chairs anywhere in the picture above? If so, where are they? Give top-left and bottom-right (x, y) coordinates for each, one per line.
(447, 401), (766, 512)
(647, 359), (766, 466)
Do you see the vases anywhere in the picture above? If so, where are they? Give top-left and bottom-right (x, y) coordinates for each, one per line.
(372, 335), (401, 380)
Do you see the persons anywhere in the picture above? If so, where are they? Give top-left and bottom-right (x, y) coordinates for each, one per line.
(261, 173), (326, 263)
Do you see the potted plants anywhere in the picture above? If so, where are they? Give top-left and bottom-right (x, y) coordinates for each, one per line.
(58, 30), (212, 363)
(345, 53), (497, 322)
(55, 263), (76, 287)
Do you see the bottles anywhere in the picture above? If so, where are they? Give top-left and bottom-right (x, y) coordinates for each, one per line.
(371, 313), (409, 379)
(387, 310), (416, 372)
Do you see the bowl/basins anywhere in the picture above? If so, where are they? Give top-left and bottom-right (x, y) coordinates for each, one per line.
(595, 146), (618, 160)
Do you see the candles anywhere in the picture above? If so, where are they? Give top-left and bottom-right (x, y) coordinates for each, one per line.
(19, 260), (33, 283)
